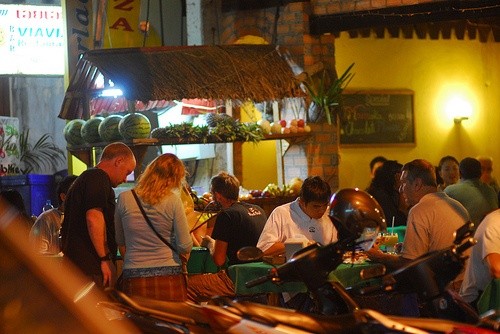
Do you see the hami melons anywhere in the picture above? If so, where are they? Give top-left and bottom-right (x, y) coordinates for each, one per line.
(288, 177), (304, 194)
(256, 120), (283, 134)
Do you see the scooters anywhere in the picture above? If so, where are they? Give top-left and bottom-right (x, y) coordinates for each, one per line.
(106, 219), (500, 334)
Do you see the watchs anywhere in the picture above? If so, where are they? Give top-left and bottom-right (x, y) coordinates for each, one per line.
(100, 252), (113, 261)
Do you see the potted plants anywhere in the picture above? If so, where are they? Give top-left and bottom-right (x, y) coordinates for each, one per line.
(302, 64), (362, 125)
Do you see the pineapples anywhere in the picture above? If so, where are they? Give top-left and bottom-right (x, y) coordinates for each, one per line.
(151, 112), (237, 139)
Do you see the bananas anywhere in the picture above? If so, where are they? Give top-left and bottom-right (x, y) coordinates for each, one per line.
(264, 183), (295, 196)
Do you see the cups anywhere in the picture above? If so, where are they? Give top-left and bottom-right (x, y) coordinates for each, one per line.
(374, 232), (382, 247)
(383, 233), (398, 255)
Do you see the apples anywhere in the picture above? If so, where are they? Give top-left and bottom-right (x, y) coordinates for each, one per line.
(237, 189), (271, 201)
(281, 119), (311, 134)
(192, 189), (221, 211)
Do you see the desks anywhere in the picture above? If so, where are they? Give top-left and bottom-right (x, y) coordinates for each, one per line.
(180, 225), (500, 312)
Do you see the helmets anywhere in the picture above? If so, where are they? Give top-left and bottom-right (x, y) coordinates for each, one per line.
(328, 187), (387, 246)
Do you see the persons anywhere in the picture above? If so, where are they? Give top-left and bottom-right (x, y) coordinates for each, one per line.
(0, 141), (500, 334)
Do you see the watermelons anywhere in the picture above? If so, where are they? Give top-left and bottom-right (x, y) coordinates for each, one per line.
(64, 112), (152, 146)
